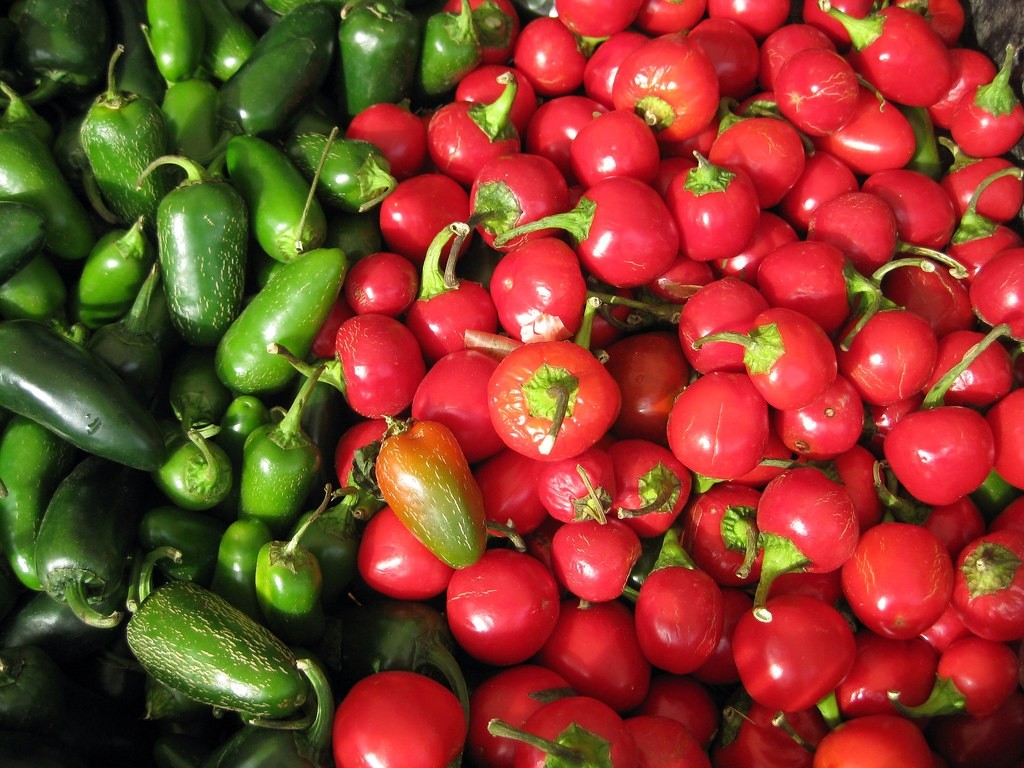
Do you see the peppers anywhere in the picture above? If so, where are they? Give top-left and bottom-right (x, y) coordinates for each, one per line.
(0, 0), (1024, 768)
